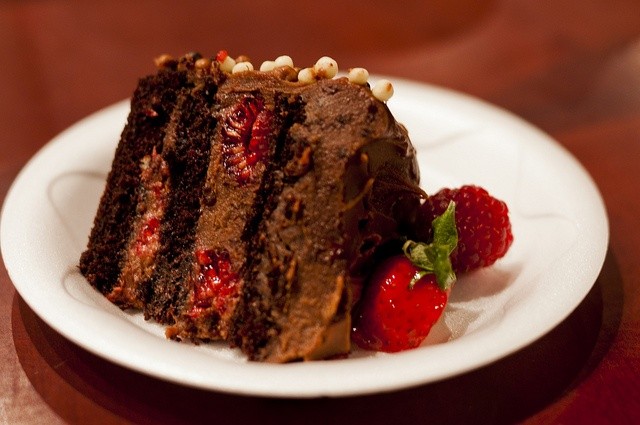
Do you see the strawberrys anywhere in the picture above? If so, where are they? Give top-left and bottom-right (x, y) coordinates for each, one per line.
(362, 251), (448, 354)
(422, 185), (513, 275)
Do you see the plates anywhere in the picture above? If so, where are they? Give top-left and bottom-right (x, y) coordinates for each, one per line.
(0, 67), (612, 399)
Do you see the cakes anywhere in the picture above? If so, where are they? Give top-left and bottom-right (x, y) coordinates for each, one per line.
(78, 50), (513, 362)
